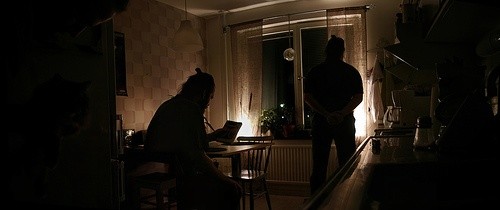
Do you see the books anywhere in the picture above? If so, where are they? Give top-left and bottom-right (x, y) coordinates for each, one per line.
(217, 121), (241, 143)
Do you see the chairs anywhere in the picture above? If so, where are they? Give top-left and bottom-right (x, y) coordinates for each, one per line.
(230, 135), (275, 210)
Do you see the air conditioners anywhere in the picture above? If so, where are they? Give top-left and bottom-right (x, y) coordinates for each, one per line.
(264, 143), (340, 186)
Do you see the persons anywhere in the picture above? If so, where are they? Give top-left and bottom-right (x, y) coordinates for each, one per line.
(305, 35), (362, 195)
(146, 67), (242, 209)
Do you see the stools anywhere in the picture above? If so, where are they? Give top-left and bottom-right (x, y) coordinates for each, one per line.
(134, 171), (180, 210)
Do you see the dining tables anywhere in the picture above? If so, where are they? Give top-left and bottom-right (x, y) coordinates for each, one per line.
(124, 142), (262, 210)
